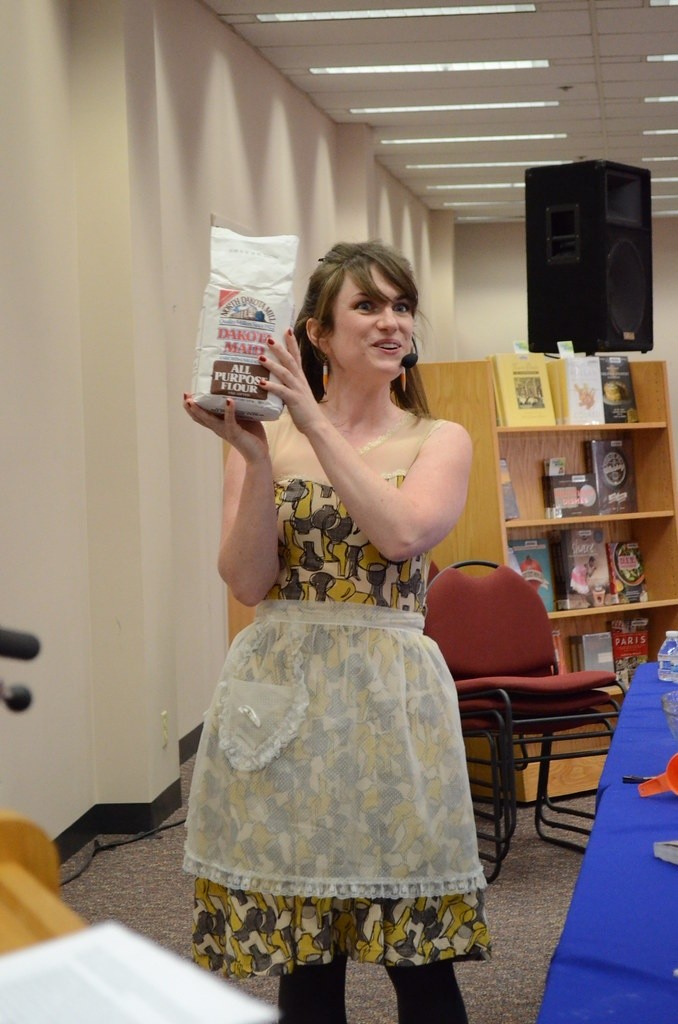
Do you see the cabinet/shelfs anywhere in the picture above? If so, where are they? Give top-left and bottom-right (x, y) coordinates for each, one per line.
(218, 358), (678, 806)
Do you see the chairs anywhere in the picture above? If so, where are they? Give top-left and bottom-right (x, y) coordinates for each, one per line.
(422, 560), (627, 886)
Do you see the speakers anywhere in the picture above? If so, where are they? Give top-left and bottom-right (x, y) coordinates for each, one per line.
(524, 159), (654, 355)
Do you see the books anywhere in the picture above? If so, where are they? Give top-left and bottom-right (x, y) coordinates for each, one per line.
(486, 352), (639, 428)
(497, 437), (638, 521)
(507, 527), (649, 613)
(550, 618), (649, 690)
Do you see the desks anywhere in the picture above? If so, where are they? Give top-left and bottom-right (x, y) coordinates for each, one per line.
(538, 662), (678, 1024)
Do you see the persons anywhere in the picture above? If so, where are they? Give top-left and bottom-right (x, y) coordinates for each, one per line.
(178, 240), (493, 1024)
